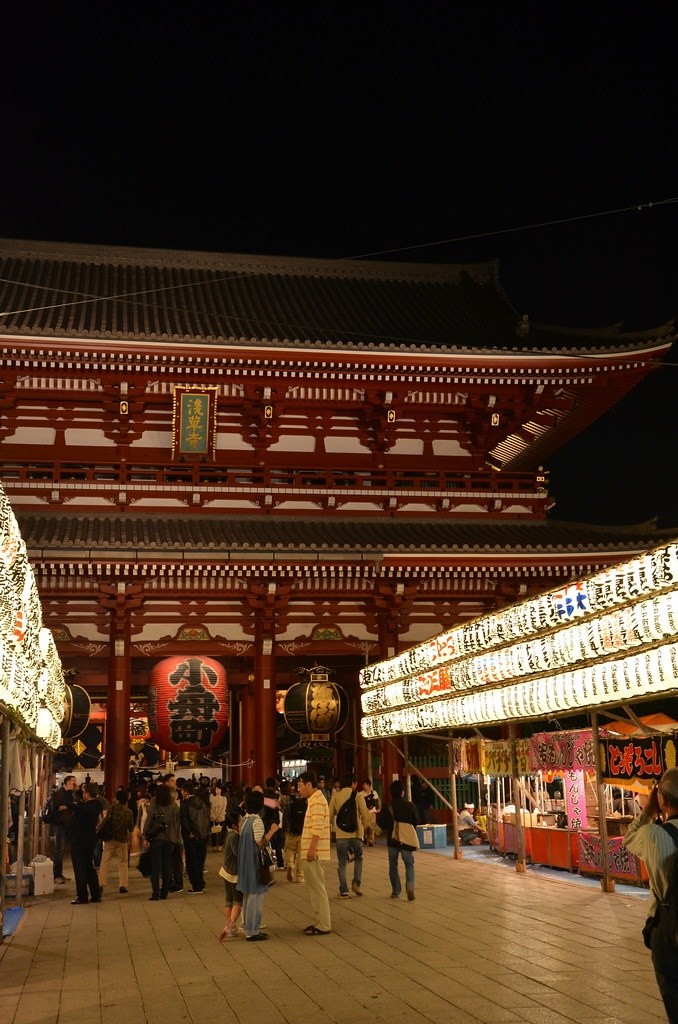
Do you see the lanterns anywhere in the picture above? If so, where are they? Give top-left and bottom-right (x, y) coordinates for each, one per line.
(281, 665), (349, 744)
(59, 669), (91, 740)
(130, 717), (151, 750)
(358, 540), (678, 743)
(0, 480), (64, 750)
(147, 655), (228, 764)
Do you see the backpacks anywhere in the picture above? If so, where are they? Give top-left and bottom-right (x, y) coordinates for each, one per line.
(287, 795), (309, 833)
(647, 822), (678, 978)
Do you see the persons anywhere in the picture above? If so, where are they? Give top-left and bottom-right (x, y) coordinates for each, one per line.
(458, 801), (485, 846)
(40, 773), (383, 904)
(418, 778), (435, 823)
(376, 780), (418, 902)
(622, 767), (678, 1024)
(297, 772), (332, 935)
(608, 793), (642, 816)
(219, 812), (244, 936)
(236, 791), (279, 940)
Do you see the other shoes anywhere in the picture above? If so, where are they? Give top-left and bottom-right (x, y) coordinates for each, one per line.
(278, 863), (305, 883)
(71, 898), (89, 904)
(119, 886), (128, 893)
(100, 887), (104, 893)
(245, 931), (270, 942)
(228, 925), (246, 938)
(88, 897), (101, 902)
(336, 890), (349, 899)
(148, 895), (167, 902)
(407, 895), (416, 902)
(215, 928), (229, 941)
(352, 885), (363, 896)
(390, 892), (400, 898)
(167, 888), (183, 895)
(185, 886), (206, 895)
(54, 874), (71, 884)
(212, 846), (222, 853)
(303, 924), (331, 936)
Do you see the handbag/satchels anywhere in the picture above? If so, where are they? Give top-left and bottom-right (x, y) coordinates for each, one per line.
(388, 801), (420, 852)
(211, 819), (224, 834)
(95, 806), (114, 842)
(335, 790), (358, 833)
(135, 851), (160, 879)
(256, 864), (279, 887)
(41, 795), (72, 824)
(145, 801), (167, 840)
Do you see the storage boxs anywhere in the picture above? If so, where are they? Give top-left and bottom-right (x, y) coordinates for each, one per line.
(5, 858), (53, 896)
(415, 824), (448, 849)
